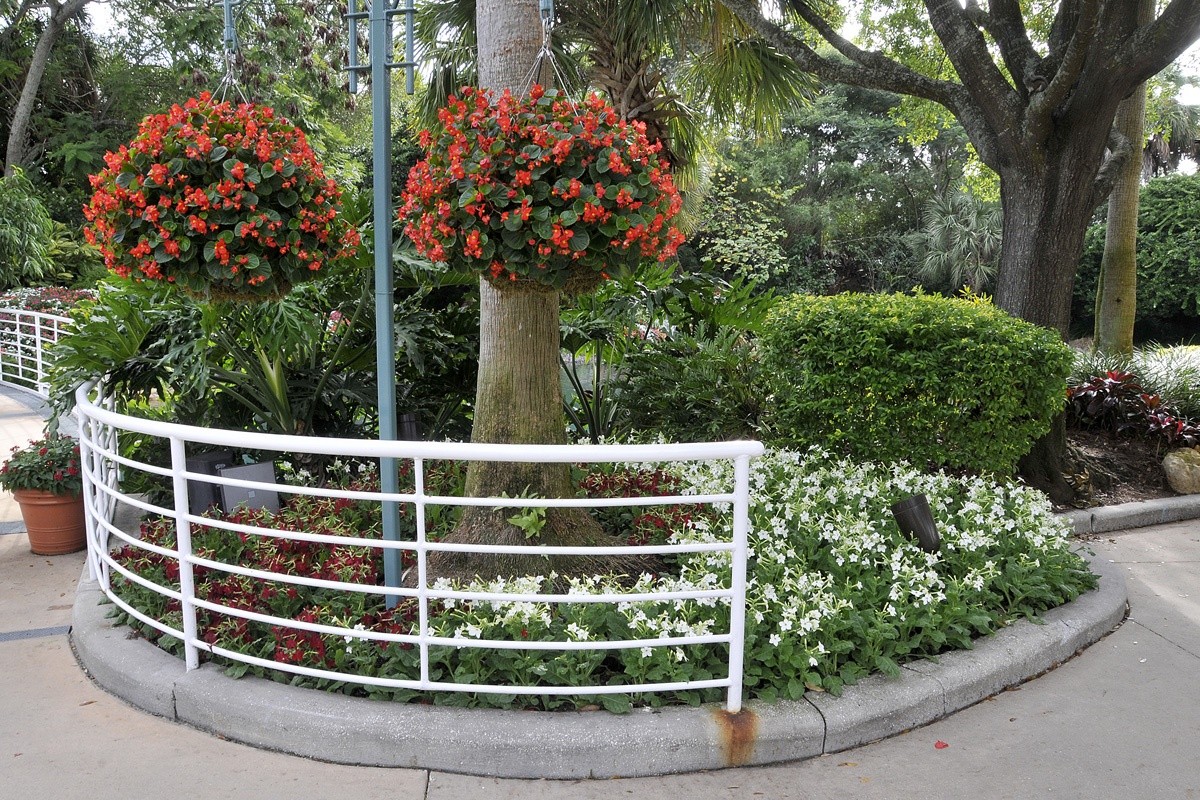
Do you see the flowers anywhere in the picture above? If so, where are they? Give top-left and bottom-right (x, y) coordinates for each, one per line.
(80, 91), (361, 293)
(0, 428), (85, 498)
(394, 74), (684, 286)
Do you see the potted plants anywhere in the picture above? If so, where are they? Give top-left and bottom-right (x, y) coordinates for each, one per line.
(33, 284), (377, 525)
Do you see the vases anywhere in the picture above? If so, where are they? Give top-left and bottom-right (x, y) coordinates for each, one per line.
(9, 487), (91, 558)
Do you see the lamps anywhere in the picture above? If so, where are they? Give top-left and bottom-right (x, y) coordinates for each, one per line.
(891, 493), (953, 575)
